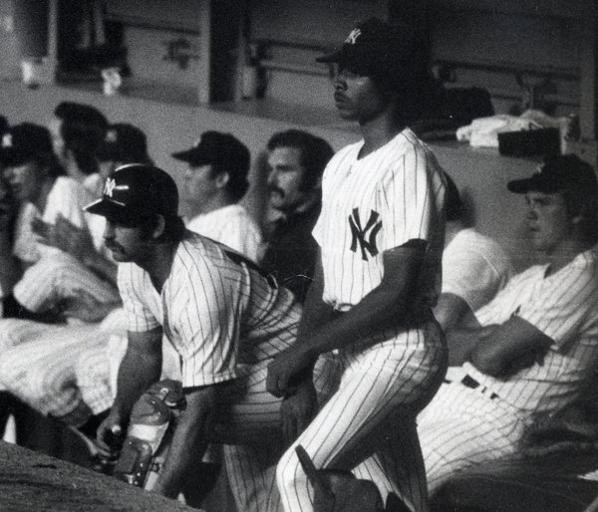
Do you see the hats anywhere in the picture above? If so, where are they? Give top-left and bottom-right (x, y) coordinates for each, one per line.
(316, 17), (417, 65)
(507, 153), (596, 195)
(170, 130), (251, 170)
(90, 123), (149, 151)
(1, 122), (52, 159)
(81, 164), (180, 217)
